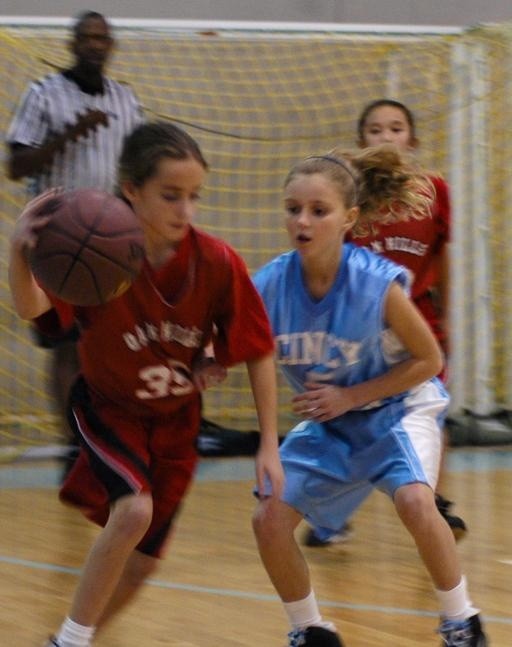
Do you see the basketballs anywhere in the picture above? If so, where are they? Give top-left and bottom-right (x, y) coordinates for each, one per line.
(26, 191), (145, 306)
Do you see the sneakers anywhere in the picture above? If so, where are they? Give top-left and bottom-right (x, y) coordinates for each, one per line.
(303, 522), (354, 544)
(433, 493), (466, 543)
(287, 625), (343, 646)
(435, 614), (486, 647)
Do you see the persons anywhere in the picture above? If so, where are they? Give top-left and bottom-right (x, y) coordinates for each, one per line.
(9, 123), (287, 647)
(303, 102), (469, 554)
(252, 140), (492, 647)
(4, 12), (152, 490)
